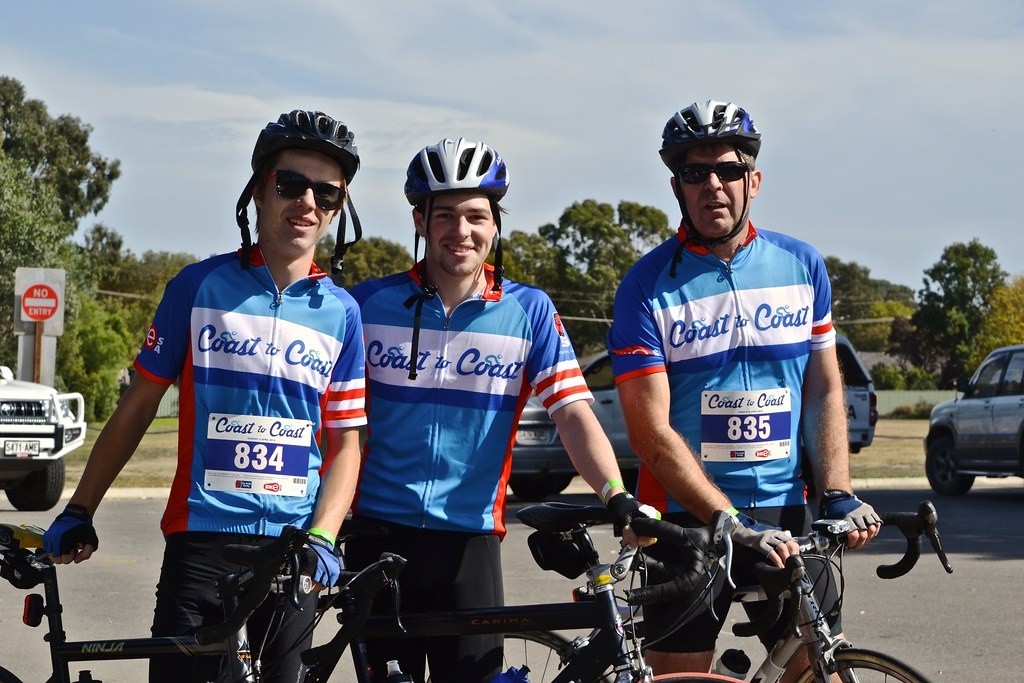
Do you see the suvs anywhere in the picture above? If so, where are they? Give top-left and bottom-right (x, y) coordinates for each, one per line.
(0, 367), (87, 512)
(923, 343), (1024, 498)
(508, 328), (880, 501)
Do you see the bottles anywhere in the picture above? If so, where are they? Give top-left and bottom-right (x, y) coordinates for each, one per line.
(71, 670), (102, 683)
(383, 660), (414, 683)
(489, 664), (531, 683)
(710, 648), (751, 680)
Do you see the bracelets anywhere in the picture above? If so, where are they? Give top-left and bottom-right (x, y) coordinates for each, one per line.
(599, 479), (628, 504)
(725, 505), (738, 519)
(308, 525), (335, 545)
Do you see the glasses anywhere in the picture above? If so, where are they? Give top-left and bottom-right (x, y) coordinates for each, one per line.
(267, 169), (346, 211)
(675, 161), (755, 184)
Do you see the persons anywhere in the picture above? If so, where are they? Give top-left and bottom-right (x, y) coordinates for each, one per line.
(609, 101), (884, 683)
(347, 137), (661, 683)
(41, 110), (369, 683)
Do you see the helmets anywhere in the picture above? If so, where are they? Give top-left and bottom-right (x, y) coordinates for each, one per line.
(404, 138), (511, 203)
(252, 109), (360, 187)
(659, 100), (763, 172)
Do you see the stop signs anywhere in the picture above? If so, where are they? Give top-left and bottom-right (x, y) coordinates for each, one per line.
(21, 284), (59, 321)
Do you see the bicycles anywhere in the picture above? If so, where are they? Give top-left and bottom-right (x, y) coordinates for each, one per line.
(217, 512), (748, 683)
(0, 521), (410, 683)
(501, 500), (955, 683)
